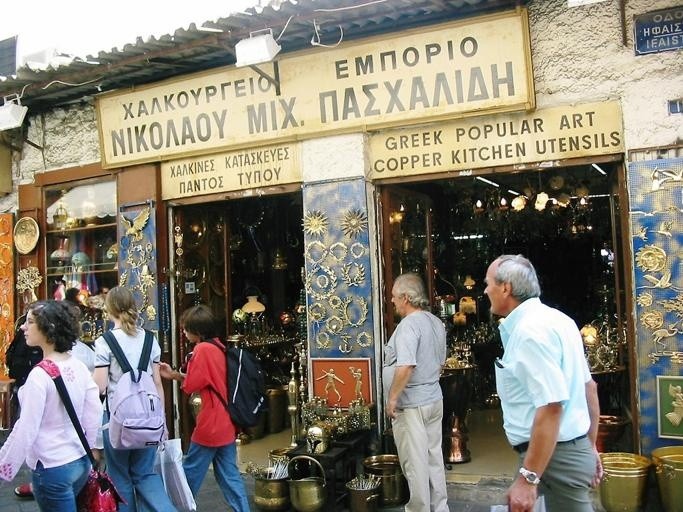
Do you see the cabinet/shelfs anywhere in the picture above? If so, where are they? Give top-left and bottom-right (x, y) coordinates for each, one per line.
(43, 214), (123, 330)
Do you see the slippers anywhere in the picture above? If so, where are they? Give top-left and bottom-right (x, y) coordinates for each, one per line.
(13, 482), (36, 500)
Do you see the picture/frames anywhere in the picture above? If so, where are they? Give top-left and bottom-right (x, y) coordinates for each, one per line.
(653, 375), (682, 441)
(307, 356), (373, 411)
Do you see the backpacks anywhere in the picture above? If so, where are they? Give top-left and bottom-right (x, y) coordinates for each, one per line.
(199, 338), (268, 427)
(5, 324), (44, 386)
(99, 327), (169, 452)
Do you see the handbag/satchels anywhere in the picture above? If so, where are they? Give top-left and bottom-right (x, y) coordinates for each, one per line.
(78, 470), (129, 512)
(153, 438), (197, 511)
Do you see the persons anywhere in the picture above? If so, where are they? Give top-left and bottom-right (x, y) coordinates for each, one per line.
(100, 287), (109, 298)
(315, 367), (341, 401)
(382, 273), (450, 512)
(160, 303), (250, 512)
(63, 289), (90, 312)
(5, 311), (42, 498)
(483, 255), (605, 512)
(0, 298), (103, 512)
(92, 285), (177, 512)
(348, 366), (363, 401)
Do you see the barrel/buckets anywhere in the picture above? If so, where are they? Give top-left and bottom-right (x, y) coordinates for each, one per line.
(361, 453), (410, 509)
(598, 452), (653, 512)
(264, 385), (285, 434)
(243, 414), (266, 440)
(254, 466), (290, 512)
(285, 455), (329, 512)
(281, 384), (296, 430)
(650, 445), (683, 512)
(346, 479), (382, 512)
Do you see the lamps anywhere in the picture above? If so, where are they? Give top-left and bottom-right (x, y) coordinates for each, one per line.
(470, 171), (600, 235)
(1, 93), (32, 132)
(228, 291), (296, 344)
(233, 26), (287, 68)
(431, 264), (483, 330)
(580, 324), (602, 369)
(49, 247), (72, 273)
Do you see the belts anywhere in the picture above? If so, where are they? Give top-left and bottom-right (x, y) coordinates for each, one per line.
(513, 435), (587, 453)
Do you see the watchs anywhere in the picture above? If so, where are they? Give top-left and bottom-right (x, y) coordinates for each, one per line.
(519, 468), (540, 485)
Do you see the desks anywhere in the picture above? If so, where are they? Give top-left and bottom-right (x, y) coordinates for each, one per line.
(288, 442), (356, 512)
(442, 364), (475, 465)
(588, 365), (626, 417)
(439, 374), (457, 471)
